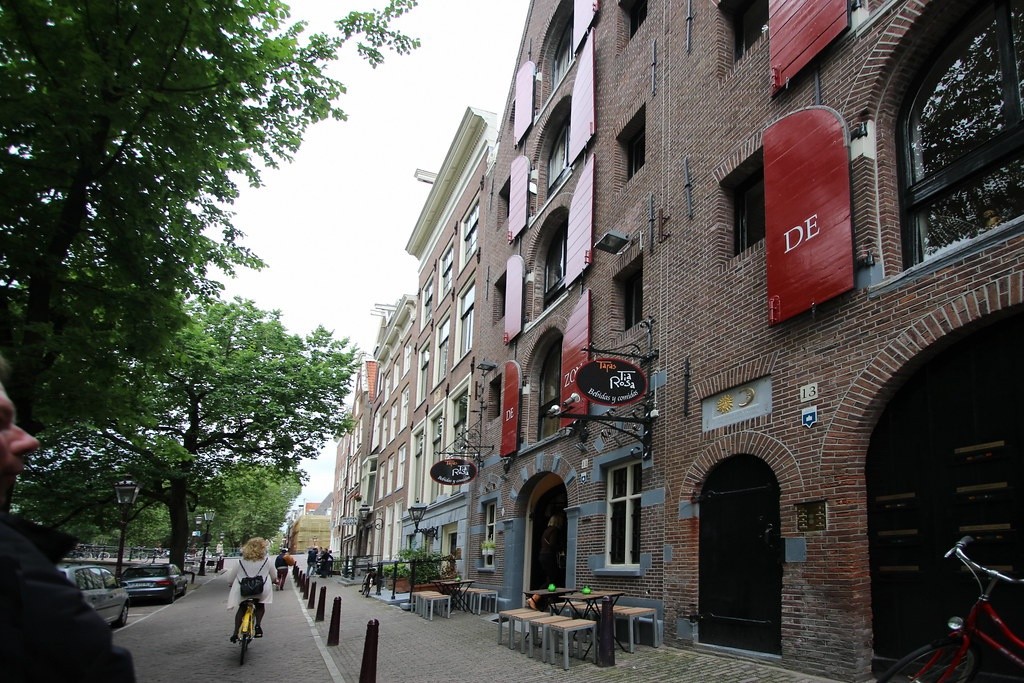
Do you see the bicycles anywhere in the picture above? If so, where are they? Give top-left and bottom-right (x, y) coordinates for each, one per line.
(876, 536), (1024, 683)
(227, 583), (278, 665)
(360, 568), (376, 598)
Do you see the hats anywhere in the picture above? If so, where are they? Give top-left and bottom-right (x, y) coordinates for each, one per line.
(281, 547), (288, 552)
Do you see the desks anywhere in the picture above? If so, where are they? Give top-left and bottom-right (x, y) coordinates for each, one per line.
(431, 579), (625, 667)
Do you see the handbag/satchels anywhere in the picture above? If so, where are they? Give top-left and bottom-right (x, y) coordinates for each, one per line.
(369, 578), (373, 588)
(283, 553), (295, 566)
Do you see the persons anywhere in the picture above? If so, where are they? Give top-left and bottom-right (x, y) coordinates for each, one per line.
(307, 547), (333, 577)
(275, 548), (295, 591)
(217, 541), (223, 560)
(526, 515), (562, 610)
(228, 538), (280, 641)
(0, 358), (137, 683)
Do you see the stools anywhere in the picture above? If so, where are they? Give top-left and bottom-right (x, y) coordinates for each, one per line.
(410, 589), (658, 670)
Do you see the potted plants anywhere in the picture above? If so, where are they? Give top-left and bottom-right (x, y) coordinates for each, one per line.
(481, 540), (488, 555)
(379, 564), (412, 593)
(486, 542), (495, 555)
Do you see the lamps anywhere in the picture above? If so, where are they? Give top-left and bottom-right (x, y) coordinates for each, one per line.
(476, 360), (497, 371)
(359, 501), (383, 530)
(593, 229), (631, 256)
(410, 498), (438, 538)
(547, 393), (580, 416)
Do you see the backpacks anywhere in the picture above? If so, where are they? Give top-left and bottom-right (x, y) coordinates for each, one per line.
(236, 557), (268, 596)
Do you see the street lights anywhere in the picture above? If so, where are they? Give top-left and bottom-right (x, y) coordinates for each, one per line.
(198, 508), (215, 576)
(113, 475), (141, 580)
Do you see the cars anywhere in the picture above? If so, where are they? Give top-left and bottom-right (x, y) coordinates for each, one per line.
(120, 562), (188, 606)
(53, 565), (132, 629)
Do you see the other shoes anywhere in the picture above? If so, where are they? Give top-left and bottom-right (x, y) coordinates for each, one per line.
(230, 634), (238, 643)
(280, 586), (283, 590)
(275, 585), (279, 591)
(255, 625), (263, 634)
